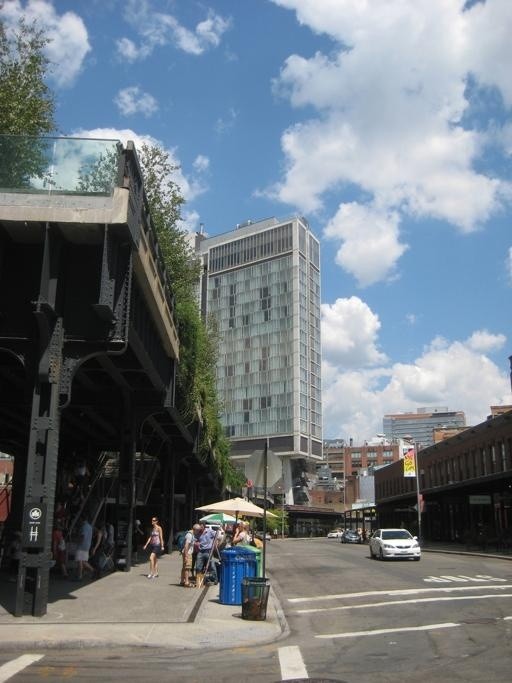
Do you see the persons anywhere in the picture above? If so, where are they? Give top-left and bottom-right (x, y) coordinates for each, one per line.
(142, 514), (166, 579)
(49, 518), (68, 581)
(131, 516), (147, 563)
(178, 520), (221, 588)
(71, 512), (116, 583)
(230, 520), (251, 548)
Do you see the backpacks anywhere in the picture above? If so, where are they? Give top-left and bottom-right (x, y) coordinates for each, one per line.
(174, 529), (194, 552)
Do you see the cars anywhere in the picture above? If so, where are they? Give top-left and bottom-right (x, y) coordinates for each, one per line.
(174, 525), (226, 548)
(328, 530), (341, 538)
(370, 528), (421, 560)
(341, 531), (361, 544)
(256, 532), (271, 541)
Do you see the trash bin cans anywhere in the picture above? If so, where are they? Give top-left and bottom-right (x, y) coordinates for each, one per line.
(218, 544), (270, 620)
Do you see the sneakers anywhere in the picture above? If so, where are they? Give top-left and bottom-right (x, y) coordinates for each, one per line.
(147, 572), (160, 579)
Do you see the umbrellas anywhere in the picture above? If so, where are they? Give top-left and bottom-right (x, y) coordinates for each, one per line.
(197, 511), (242, 525)
(193, 496), (280, 522)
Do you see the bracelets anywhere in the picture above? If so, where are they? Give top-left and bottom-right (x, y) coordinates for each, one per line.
(161, 546), (164, 548)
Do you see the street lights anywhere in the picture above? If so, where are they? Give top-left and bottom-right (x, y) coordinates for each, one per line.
(376, 433), (424, 547)
